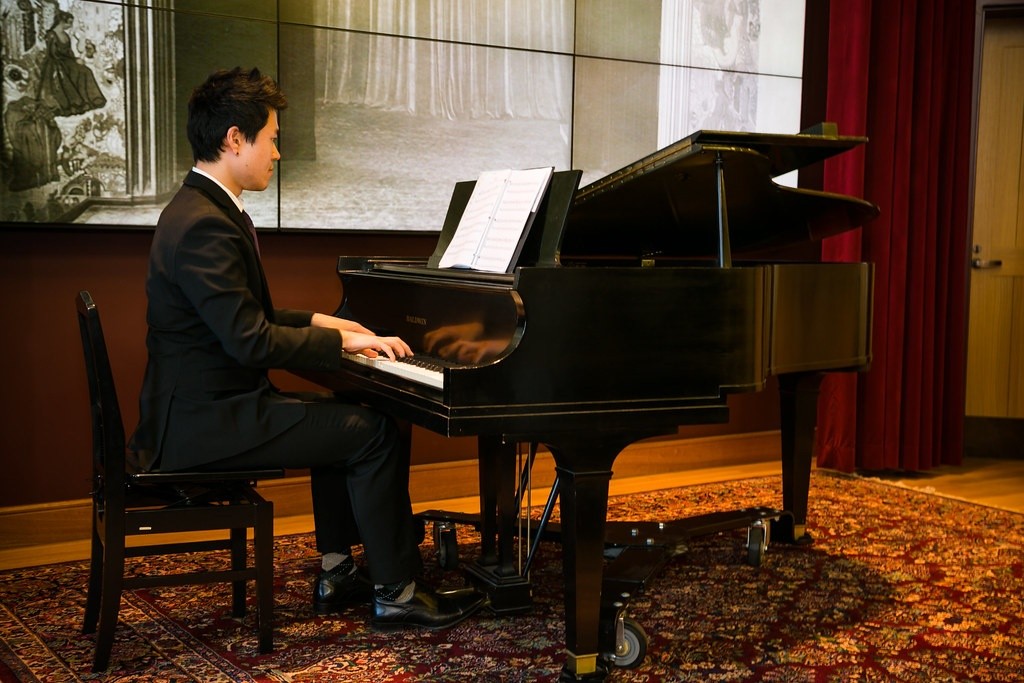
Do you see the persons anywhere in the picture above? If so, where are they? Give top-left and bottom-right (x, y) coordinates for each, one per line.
(125, 63), (486, 633)
(422, 316), (510, 364)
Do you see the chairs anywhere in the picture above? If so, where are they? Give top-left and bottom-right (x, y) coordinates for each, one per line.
(75, 290), (275, 672)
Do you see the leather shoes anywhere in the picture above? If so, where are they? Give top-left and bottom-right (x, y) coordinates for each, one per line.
(372, 580), (489, 629)
(313, 564), (376, 613)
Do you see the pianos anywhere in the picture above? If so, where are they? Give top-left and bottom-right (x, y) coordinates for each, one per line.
(284, 129), (884, 683)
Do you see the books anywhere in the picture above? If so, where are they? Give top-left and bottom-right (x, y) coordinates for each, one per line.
(437, 168), (554, 271)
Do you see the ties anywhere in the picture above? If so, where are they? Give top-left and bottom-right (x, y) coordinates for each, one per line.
(241, 211), (262, 262)
